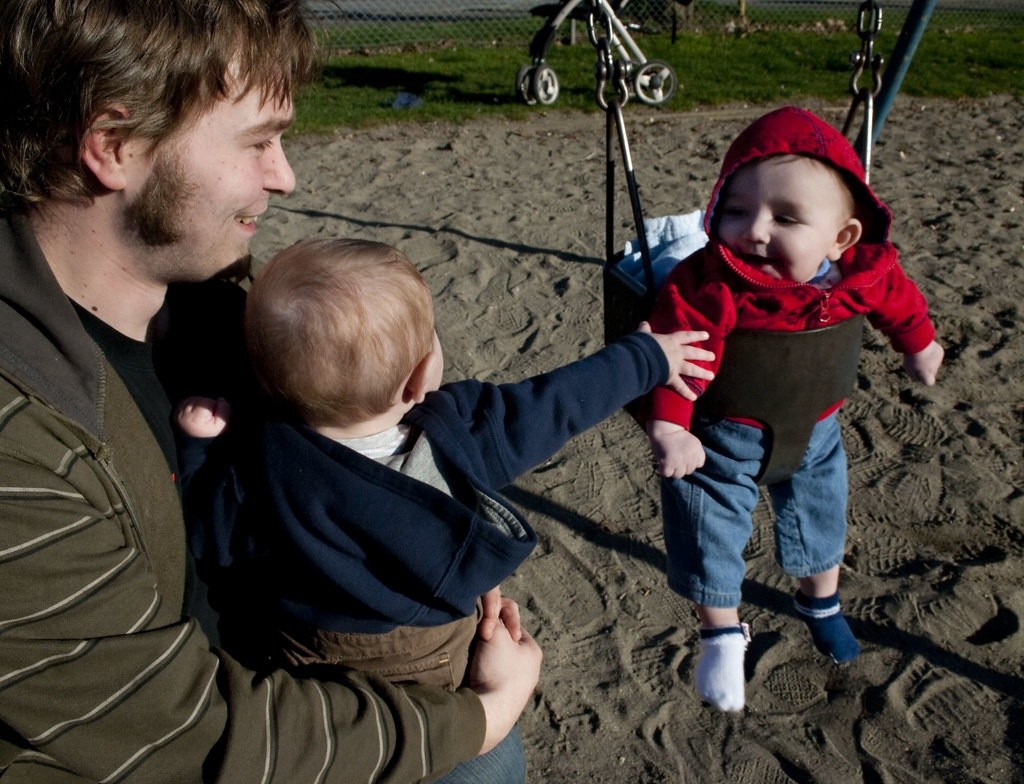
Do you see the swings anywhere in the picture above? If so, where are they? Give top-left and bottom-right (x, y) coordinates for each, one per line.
(586, 0), (890, 485)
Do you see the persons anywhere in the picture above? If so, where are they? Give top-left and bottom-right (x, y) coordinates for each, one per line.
(1, 1), (544, 784)
(644, 105), (944, 714)
(177, 236), (715, 692)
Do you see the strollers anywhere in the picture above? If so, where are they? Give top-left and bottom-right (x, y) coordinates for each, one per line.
(516, 0), (677, 105)
